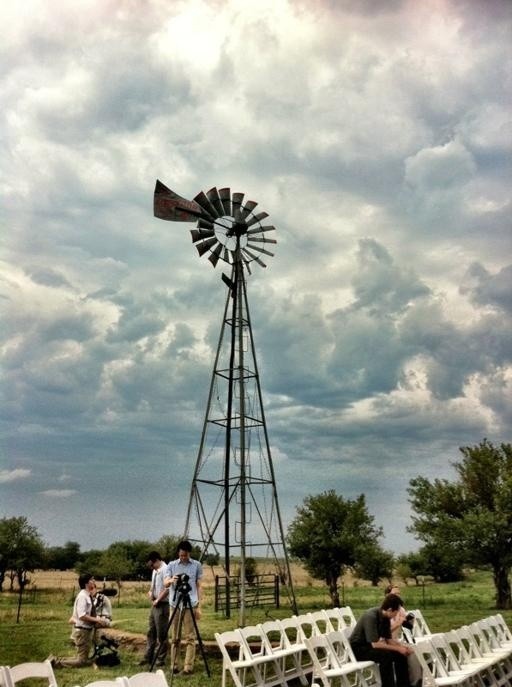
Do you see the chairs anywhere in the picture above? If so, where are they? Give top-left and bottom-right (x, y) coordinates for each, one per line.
(85, 669), (167, 687)
(1, 660), (58, 686)
(214, 606), (512, 687)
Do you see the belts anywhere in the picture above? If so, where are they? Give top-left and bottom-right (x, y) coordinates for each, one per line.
(154, 598), (169, 604)
(75, 625), (93, 631)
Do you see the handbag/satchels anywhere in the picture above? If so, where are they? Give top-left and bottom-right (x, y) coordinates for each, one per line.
(94, 652), (121, 667)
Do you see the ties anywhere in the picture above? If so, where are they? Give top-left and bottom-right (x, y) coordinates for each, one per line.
(153, 573), (157, 593)
(89, 595), (97, 618)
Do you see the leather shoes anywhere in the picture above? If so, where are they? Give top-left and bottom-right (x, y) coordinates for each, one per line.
(173, 663), (180, 674)
(139, 660), (153, 665)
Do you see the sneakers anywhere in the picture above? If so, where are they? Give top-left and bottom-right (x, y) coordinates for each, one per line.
(156, 662), (166, 667)
(183, 663), (194, 675)
(43, 653), (57, 667)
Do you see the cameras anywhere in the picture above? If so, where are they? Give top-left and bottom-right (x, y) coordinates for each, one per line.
(402, 613), (415, 630)
(175, 573), (190, 587)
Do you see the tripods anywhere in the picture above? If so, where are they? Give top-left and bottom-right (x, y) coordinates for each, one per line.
(150, 595), (211, 687)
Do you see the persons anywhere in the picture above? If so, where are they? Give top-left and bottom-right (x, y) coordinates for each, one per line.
(351, 595), (414, 687)
(138, 551), (169, 667)
(163, 541), (203, 675)
(384, 584), (423, 687)
(68, 582), (111, 629)
(43, 573), (105, 667)
